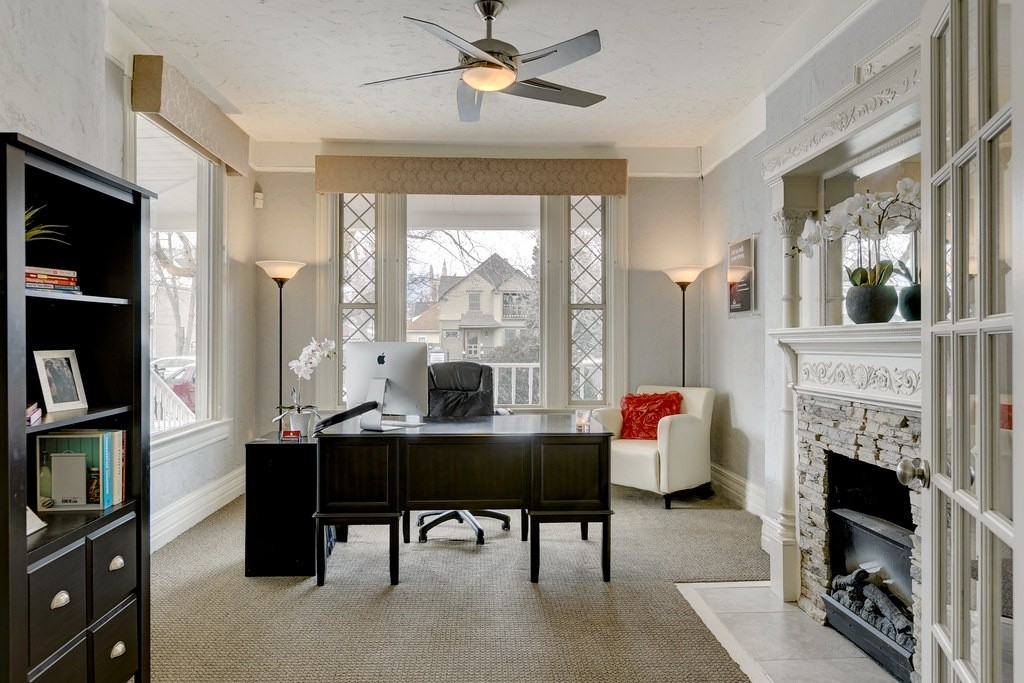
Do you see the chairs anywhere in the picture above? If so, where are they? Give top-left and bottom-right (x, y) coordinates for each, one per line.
(417, 363), (510, 545)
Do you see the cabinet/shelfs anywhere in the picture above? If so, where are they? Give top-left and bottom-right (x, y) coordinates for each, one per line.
(0, 132), (157, 683)
(245, 432), (335, 577)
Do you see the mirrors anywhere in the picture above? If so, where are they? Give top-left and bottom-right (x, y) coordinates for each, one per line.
(822, 126), (922, 326)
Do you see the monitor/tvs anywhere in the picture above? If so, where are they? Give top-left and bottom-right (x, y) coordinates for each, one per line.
(342, 340), (429, 431)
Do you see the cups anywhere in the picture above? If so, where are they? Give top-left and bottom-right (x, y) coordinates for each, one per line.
(576, 410), (591, 430)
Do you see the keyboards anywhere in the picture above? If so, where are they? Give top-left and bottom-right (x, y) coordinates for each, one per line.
(380, 420), (427, 427)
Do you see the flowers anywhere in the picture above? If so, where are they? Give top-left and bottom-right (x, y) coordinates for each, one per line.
(784, 177), (921, 286)
(271, 337), (335, 422)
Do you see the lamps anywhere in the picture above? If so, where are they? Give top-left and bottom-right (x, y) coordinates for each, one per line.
(462, 62), (516, 91)
(662, 265), (706, 387)
(255, 260), (306, 432)
(727, 265), (752, 290)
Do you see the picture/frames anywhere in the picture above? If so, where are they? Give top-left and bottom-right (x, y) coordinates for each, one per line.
(727, 232), (761, 319)
(33, 350), (89, 412)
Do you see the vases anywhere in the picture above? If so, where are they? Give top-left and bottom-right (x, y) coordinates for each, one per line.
(899, 284), (921, 321)
(290, 411), (312, 436)
(845, 286), (899, 324)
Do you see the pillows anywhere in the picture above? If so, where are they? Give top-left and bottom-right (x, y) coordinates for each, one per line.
(619, 390), (683, 440)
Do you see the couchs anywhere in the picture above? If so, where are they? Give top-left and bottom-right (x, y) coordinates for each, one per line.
(592, 385), (715, 509)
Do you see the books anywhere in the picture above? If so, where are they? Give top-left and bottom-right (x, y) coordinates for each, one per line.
(26, 401), (42, 425)
(37, 428), (126, 510)
(25, 266), (82, 295)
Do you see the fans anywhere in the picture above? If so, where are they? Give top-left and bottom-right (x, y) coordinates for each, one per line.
(358, 0), (607, 122)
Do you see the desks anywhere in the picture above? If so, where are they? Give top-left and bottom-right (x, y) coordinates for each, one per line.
(312, 413), (615, 586)
